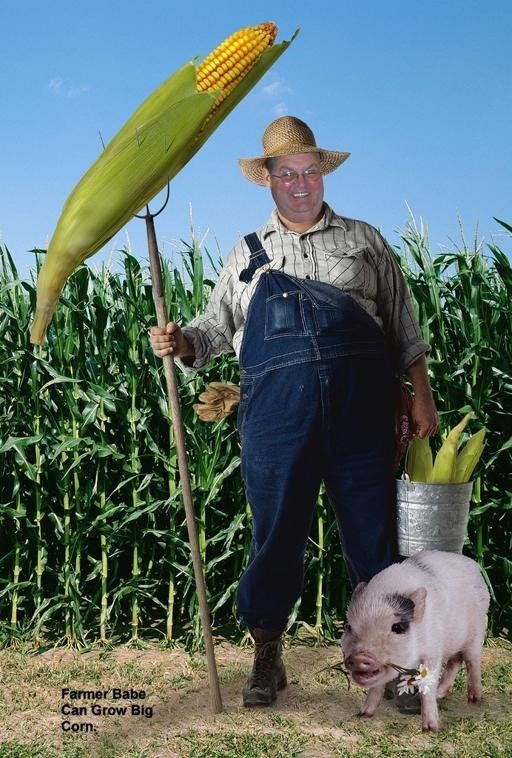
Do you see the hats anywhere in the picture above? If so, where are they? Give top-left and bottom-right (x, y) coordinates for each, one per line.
(239, 115), (351, 187)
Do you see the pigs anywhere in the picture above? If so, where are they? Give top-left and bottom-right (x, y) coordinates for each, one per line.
(340, 548), (501, 737)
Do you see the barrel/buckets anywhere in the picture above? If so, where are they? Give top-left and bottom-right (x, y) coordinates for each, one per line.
(396, 476), (474, 557)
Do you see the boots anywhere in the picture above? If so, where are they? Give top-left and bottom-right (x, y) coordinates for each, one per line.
(385, 671), (421, 714)
(242, 634), (287, 706)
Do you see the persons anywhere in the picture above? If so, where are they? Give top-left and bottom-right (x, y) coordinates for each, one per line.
(147, 114), (443, 706)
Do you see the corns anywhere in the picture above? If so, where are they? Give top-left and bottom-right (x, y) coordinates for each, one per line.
(406, 410), (489, 485)
(30, 21), (302, 343)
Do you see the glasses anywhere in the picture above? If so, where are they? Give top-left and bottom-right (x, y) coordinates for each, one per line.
(270, 169), (319, 183)
